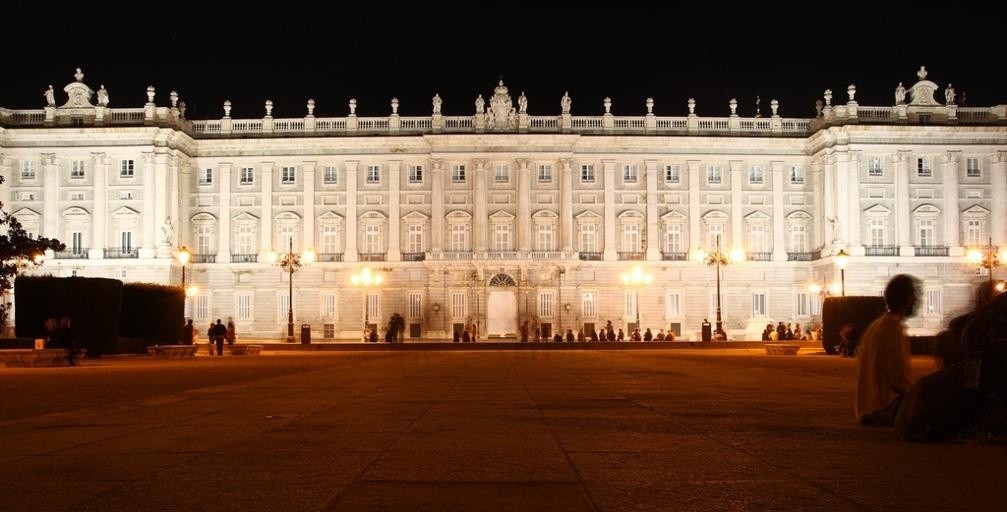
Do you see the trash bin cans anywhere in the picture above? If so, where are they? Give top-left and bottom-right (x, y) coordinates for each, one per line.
(301, 324), (309, 344)
(701, 322), (710, 342)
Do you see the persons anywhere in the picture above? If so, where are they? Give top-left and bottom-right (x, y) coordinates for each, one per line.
(181, 316), (235, 356)
(432, 93), (442, 113)
(44, 67), (109, 106)
(474, 80), (528, 114)
(560, 91), (572, 113)
(46, 317), (80, 365)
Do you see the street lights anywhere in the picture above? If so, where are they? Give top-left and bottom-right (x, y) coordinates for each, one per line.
(620, 267), (652, 330)
(265, 236), (315, 344)
(968, 237), (1006, 302)
(177, 245), (190, 288)
(693, 232), (745, 342)
(350, 266), (384, 342)
(834, 249), (848, 295)
(3, 250), (46, 276)
(808, 274), (837, 299)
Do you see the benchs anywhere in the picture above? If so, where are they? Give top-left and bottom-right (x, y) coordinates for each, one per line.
(762, 343), (800, 355)
(146, 344), (200, 360)
(0, 348), (86, 367)
(227, 344), (262, 355)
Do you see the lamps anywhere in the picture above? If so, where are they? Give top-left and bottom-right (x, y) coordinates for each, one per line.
(431, 301), (441, 315)
(564, 302), (571, 314)
